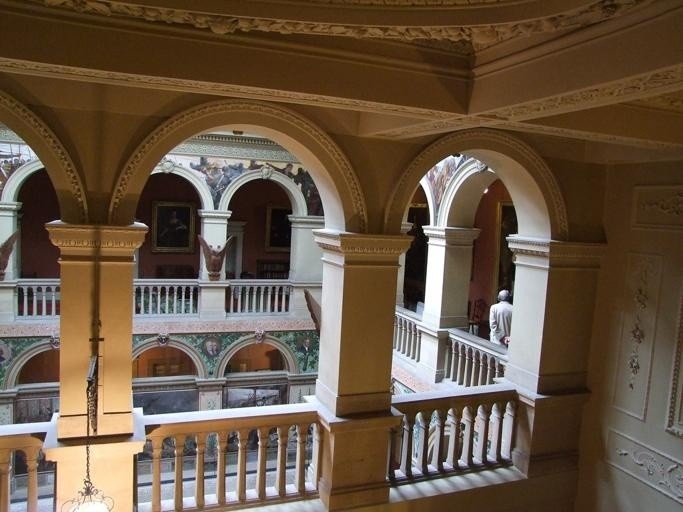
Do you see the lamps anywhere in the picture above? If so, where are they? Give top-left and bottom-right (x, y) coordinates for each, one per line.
(61, 354), (114, 512)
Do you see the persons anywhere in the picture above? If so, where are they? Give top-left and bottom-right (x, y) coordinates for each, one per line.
(157, 209), (187, 244)
(488, 289), (512, 347)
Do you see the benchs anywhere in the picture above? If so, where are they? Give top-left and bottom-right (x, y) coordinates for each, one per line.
(255, 259), (290, 299)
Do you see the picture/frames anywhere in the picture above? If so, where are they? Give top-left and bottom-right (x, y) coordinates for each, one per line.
(265, 201), (294, 254)
(491, 200), (519, 306)
(150, 200), (196, 254)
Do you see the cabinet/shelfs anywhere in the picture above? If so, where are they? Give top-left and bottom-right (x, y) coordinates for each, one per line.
(154, 265), (197, 299)
